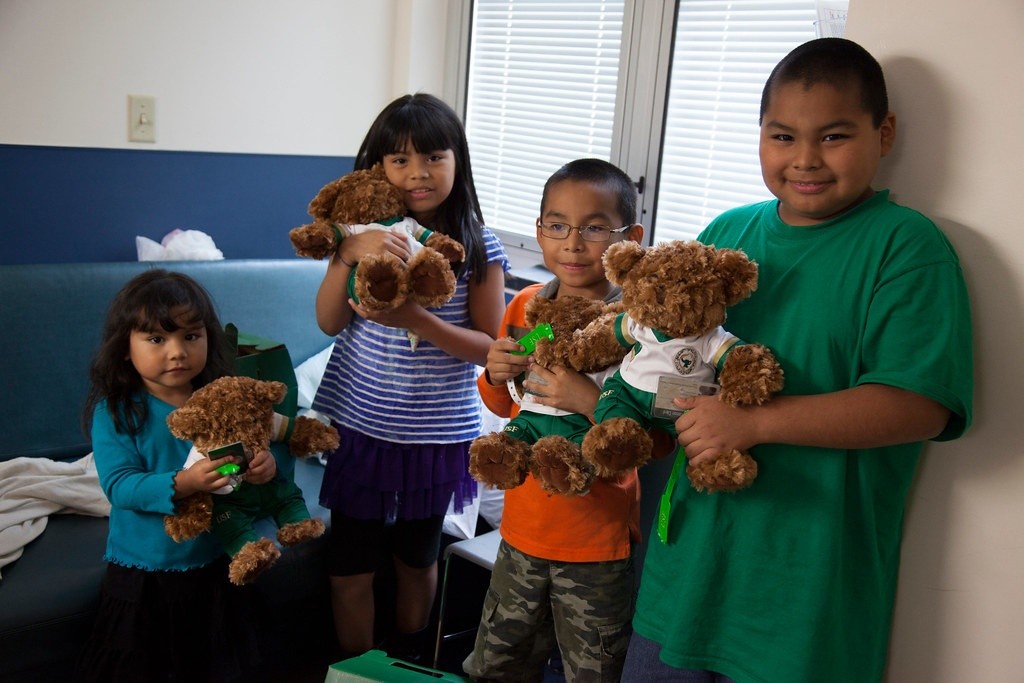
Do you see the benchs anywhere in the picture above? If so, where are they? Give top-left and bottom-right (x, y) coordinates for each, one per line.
(0, 260), (337, 683)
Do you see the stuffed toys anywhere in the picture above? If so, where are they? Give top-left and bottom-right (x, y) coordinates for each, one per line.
(468, 240), (785, 497)
(164, 376), (340, 584)
(290, 161), (465, 314)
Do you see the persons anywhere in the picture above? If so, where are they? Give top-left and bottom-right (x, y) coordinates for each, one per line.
(74, 270), (297, 683)
(620, 38), (975, 683)
(463, 158), (647, 683)
(311, 93), (507, 658)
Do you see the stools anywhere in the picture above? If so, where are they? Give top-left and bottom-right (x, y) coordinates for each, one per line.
(434, 528), (565, 683)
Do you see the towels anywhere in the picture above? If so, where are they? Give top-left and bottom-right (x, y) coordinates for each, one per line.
(1, 450), (112, 569)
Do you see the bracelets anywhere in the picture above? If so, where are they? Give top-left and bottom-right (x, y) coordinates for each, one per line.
(336, 248), (355, 270)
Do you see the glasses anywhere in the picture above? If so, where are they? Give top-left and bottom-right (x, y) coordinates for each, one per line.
(541, 222), (634, 242)
